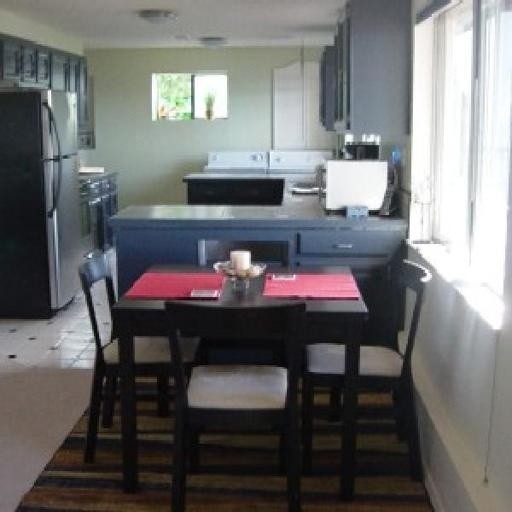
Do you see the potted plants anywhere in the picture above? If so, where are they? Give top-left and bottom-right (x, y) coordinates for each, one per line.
(204, 94), (214, 119)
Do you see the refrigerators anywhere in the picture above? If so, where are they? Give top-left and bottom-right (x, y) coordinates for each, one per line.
(0, 87), (82, 319)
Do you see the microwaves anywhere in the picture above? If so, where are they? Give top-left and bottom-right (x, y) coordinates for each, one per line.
(318, 160), (389, 216)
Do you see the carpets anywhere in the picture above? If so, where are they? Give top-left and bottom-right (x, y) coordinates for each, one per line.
(14, 383), (436, 511)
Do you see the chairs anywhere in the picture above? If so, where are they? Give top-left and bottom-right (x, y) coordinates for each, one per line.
(164, 300), (310, 512)
(188, 239), (291, 358)
(78, 259), (206, 469)
(277, 262), (431, 473)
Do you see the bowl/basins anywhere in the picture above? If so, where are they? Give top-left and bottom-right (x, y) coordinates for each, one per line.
(214, 260), (266, 300)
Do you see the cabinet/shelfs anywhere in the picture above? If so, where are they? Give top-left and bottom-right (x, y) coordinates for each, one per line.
(0, 35), (75, 91)
(320, 0), (411, 137)
(76, 53), (96, 149)
(77, 172), (118, 255)
(295, 228), (407, 330)
(117, 226), (197, 301)
